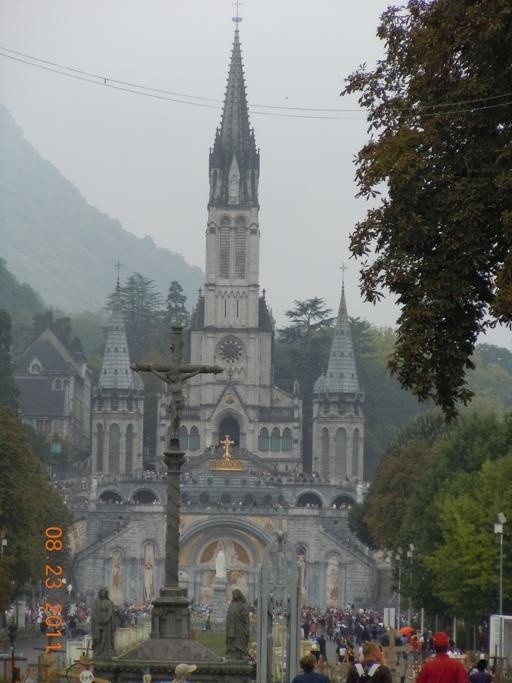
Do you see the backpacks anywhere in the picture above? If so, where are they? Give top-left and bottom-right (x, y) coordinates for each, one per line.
(353, 663), (382, 682)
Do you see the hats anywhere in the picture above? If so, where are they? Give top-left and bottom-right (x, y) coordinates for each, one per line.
(175, 664), (197, 674)
(433, 632), (449, 647)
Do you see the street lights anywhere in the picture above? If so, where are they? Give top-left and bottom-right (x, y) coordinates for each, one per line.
(495, 513), (507, 616)
(61, 578), (72, 617)
(386, 544), (415, 628)
